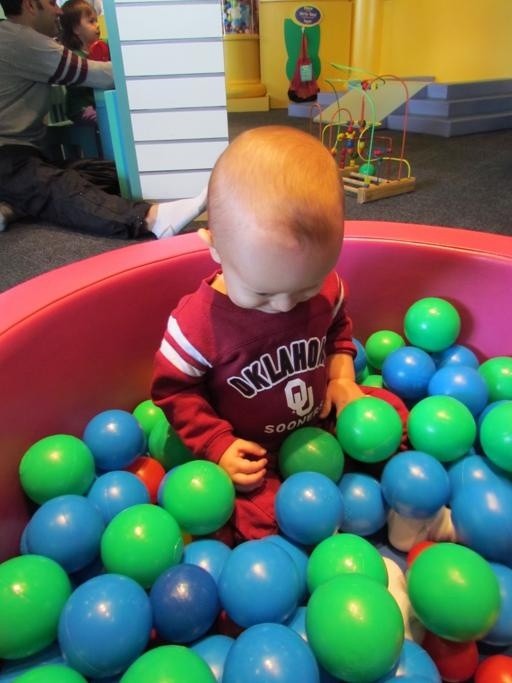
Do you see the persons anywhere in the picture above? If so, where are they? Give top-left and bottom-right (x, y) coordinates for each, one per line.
(1, 1), (210, 241)
(59, 1), (110, 123)
(148, 125), (462, 645)
